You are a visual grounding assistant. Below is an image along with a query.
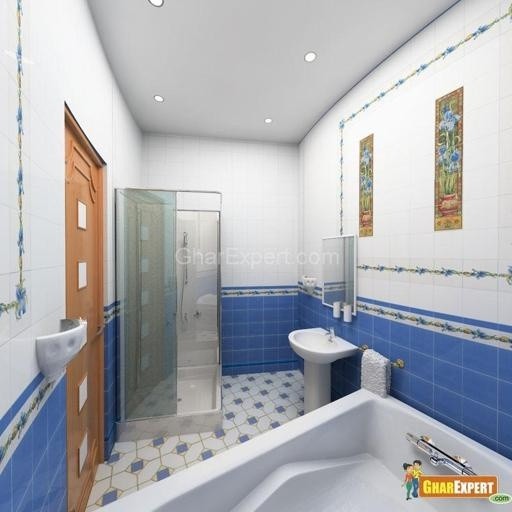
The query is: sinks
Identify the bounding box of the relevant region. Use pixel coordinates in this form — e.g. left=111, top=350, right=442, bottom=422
left=287, top=327, right=359, bottom=365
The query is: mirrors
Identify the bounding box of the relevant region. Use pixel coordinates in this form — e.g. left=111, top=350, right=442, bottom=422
left=321, top=235, right=358, bottom=317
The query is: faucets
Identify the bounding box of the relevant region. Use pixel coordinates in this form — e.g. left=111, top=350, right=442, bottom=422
left=325, top=326, right=335, bottom=342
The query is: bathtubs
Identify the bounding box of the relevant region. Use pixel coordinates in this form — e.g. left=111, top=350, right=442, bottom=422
left=93, top=389, right=512, bottom=512
left=196, top=293, right=218, bottom=329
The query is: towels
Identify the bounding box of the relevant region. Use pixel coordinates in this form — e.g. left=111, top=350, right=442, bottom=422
left=361, top=349, right=392, bottom=398
